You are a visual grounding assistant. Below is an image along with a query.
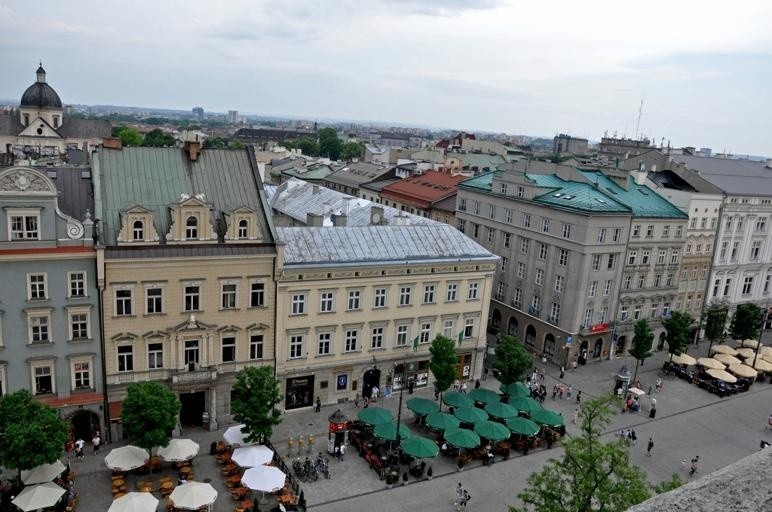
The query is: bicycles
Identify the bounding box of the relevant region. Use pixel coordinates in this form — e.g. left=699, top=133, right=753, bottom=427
left=292, top=451, right=332, bottom=483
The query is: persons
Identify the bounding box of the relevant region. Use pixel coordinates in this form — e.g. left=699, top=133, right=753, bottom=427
left=316, top=352, right=665, bottom=512
left=67, top=435, right=101, bottom=461
left=680, top=453, right=701, bottom=477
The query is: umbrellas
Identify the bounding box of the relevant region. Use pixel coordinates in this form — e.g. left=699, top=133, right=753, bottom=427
left=21, top=458, right=68, bottom=485
left=241, top=465, right=287, bottom=500
left=169, top=480, right=218, bottom=510
left=231, top=444, right=274, bottom=468
left=107, top=491, right=160, bottom=512
left=667, top=335, right=772, bottom=384
left=157, top=438, right=200, bottom=463
left=104, top=445, right=150, bottom=471
left=11, top=481, right=68, bottom=512
left=223, top=423, right=264, bottom=447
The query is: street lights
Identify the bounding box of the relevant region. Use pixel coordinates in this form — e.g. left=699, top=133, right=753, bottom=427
left=393, top=359, right=417, bottom=455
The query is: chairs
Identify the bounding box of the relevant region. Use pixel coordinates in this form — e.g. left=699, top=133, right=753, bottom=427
left=60, top=464, right=80, bottom=512
left=434, top=427, right=560, bottom=466
left=111, top=441, right=307, bottom=512
left=346, top=420, right=432, bottom=485
